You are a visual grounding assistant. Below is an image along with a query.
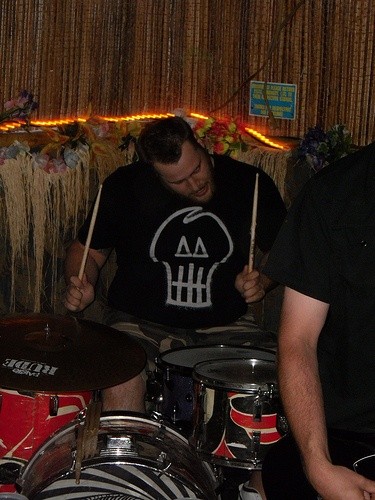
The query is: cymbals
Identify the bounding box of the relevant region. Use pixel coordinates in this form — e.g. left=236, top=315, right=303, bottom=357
left=0, top=313, right=147, bottom=391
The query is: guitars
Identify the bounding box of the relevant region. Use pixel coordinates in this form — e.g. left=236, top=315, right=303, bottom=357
left=261, top=427, right=375, bottom=500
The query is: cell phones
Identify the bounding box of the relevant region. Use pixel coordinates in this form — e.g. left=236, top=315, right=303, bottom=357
left=353, top=454, right=375, bottom=482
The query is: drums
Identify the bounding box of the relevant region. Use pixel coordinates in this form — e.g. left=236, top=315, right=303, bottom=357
left=191, top=358, right=291, bottom=470
left=18, top=410, right=221, bottom=500
left=0, top=389, right=93, bottom=462
left=150, top=345, right=278, bottom=435
left=0, top=456, right=30, bottom=492
left=0, top=492, right=30, bottom=500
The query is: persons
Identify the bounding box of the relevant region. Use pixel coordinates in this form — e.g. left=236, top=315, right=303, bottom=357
left=61, top=116, right=288, bottom=500
left=255, top=140, right=375, bottom=500
